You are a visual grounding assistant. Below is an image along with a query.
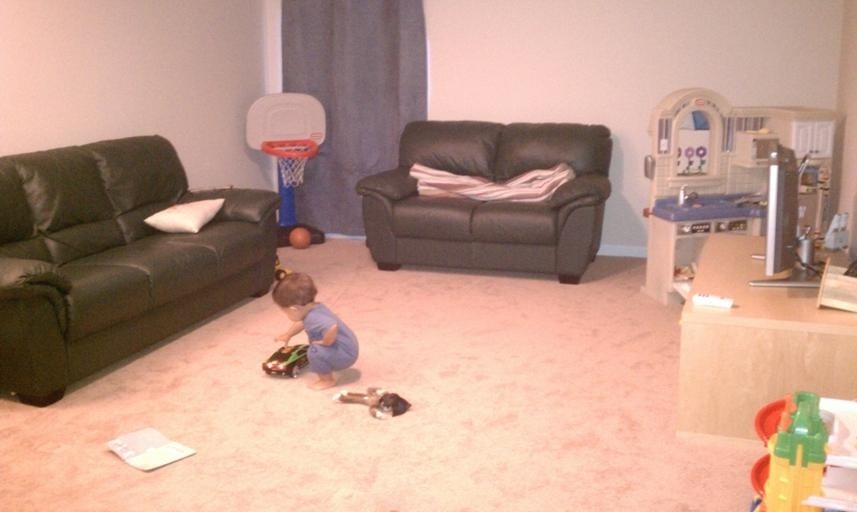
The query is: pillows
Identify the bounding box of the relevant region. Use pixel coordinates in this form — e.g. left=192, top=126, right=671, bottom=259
left=139, top=195, right=228, bottom=235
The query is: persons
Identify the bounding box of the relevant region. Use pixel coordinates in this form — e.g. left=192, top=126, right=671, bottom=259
left=333, top=384, right=411, bottom=420
left=271, top=272, right=360, bottom=391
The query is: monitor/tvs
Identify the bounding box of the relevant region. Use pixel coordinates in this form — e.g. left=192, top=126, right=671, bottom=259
left=749, top=143, right=819, bottom=288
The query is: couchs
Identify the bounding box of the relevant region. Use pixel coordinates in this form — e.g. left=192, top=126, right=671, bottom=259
left=355, top=121, right=614, bottom=284
left=0, top=130, right=283, bottom=408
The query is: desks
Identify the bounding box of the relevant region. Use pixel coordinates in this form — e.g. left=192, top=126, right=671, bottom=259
left=673, top=229, right=856, bottom=445
